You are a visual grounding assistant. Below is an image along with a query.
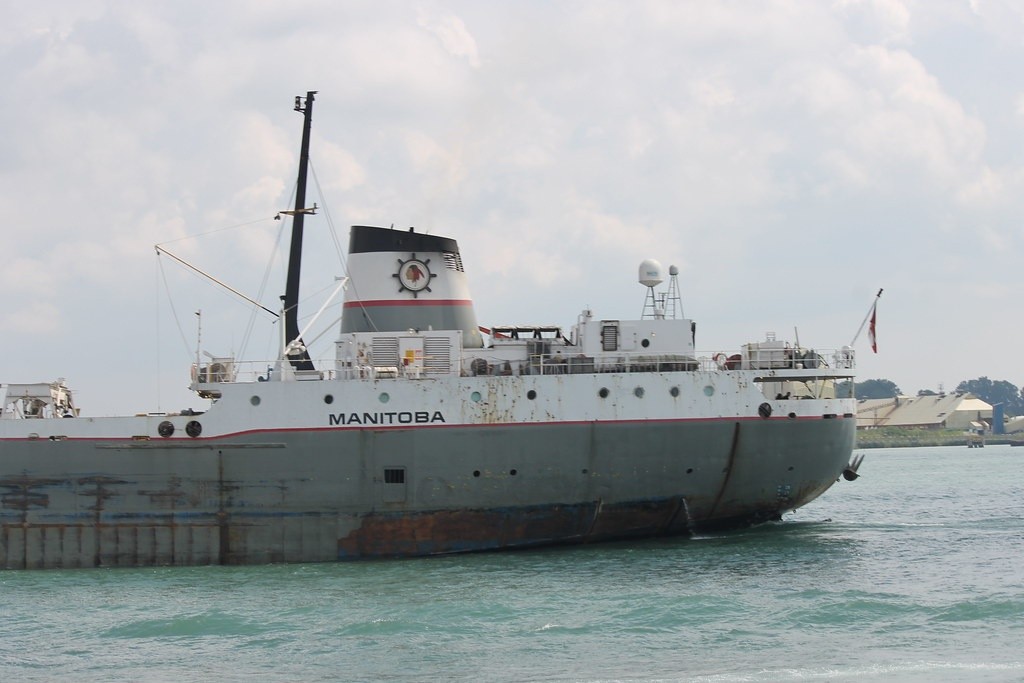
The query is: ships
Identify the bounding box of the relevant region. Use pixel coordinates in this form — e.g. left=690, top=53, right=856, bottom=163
left=0, top=91, right=886, bottom=571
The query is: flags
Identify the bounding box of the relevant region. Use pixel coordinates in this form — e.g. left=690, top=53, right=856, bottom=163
left=868, top=302, right=877, bottom=354
